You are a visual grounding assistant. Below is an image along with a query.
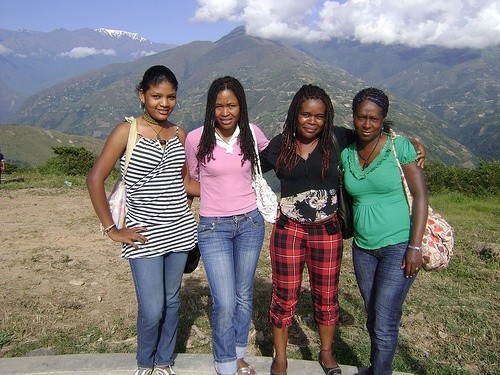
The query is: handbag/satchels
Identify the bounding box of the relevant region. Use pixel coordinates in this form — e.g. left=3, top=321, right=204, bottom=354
left=251, top=174, right=279, bottom=224
left=100, top=179, right=126, bottom=237
left=337, top=170, right=354, bottom=240
left=407, top=197, right=454, bottom=273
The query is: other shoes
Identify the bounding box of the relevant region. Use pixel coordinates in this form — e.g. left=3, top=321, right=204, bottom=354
left=152, top=365, right=176, bottom=375
left=130, top=369, right=153, bottom=375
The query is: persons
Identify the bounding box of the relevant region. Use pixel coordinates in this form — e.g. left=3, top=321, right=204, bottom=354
left=342, top=88, right=429, bottom=375
left=254, top=84, right=425, bottom=375
left=184, top=75, right=270, bottom=375
left=87, top=65, right=199, bottom=375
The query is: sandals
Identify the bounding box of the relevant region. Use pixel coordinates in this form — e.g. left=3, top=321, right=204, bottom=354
left=233, top=364, right=253, bottom=375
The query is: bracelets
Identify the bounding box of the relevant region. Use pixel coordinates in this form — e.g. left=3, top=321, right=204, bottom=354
left=408, top=245, right=424, bottom=251
left=103, top=224, right=116, bottom=232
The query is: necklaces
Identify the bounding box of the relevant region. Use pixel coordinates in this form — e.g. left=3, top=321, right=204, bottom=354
left=146, top=121, right=165, bottom=140
left=356, top=132, right=382, bottom=170
left=142, top=112, right=159, bottom=124
left=301, top=144, right=316, bottom=156
left=215, top=128, right=233, bottom=143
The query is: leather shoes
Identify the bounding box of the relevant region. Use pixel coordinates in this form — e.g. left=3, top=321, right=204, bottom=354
left=271, top=359, right=288, bottom=375
left=318, top=352, right=342, bottom=375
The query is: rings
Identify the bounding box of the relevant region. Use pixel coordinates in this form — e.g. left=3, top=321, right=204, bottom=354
left=416, top=268, right=418, bottom=270
